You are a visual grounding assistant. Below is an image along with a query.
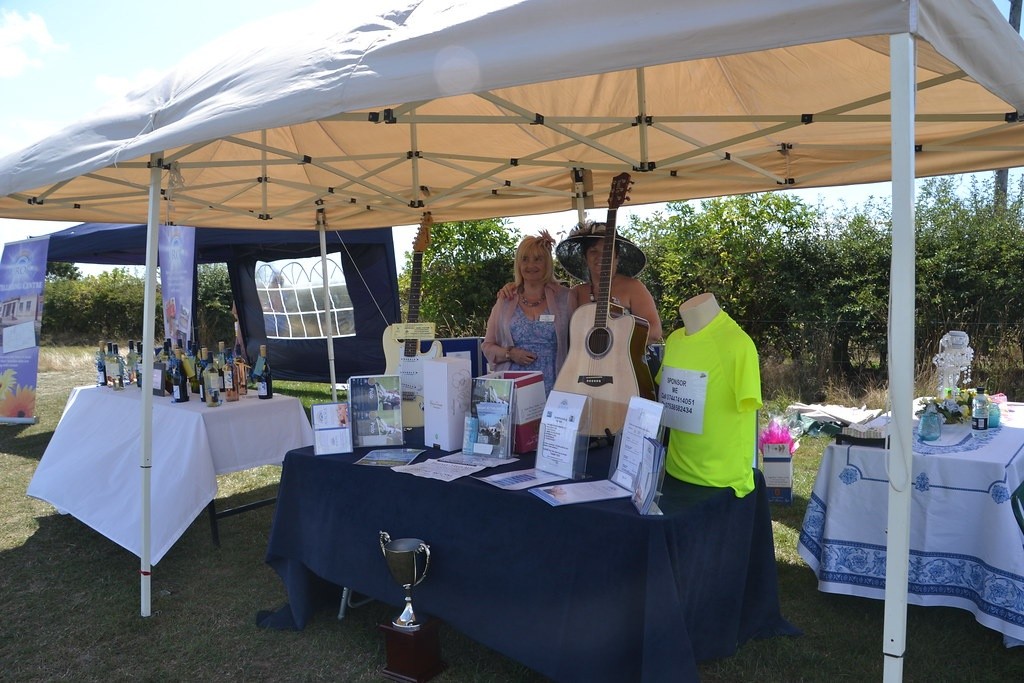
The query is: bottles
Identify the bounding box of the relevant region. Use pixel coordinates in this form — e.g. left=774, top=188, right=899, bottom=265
left=98, top=340, right=272, bottom=408
left=972, top=387, right=988, bottom=437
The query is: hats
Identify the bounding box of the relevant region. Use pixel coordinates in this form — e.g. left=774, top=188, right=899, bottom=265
left=555, top=220, right=646, bottom=282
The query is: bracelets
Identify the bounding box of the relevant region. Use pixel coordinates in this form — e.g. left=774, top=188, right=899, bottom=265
left=505, top=346, right=514, bottom=361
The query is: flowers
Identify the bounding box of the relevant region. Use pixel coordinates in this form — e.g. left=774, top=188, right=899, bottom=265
left=919, top=389, right=978, bottom=422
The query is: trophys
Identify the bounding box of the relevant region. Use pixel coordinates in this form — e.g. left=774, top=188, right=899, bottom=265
left=379, top=531, right=445, bottom=683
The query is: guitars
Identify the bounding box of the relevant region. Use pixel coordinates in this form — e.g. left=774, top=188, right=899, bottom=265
left=548, top=171, right=657, bottom=442
left=381, top=208, right=443, bottom=431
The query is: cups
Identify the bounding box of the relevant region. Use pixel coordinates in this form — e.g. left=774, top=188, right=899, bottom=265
left=988, top=403, right=1000, bottom=428
left=918, top=415, right=941, bottom=440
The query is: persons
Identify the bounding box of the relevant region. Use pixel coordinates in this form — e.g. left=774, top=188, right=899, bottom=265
left=556, top=222, right=662, bottom=345
left=480, top=236, right=579, bottom=401
left=0, top=0, right=1024, bottom=683
left=654, top=293, right=763, bottom=503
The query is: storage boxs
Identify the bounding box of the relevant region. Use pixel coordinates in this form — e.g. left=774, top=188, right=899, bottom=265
left=422, top=358, right=472, bottom=451
left=760, top=438, right=800, bottom=505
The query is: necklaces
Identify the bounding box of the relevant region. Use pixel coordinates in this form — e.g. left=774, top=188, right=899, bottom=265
left=590, top=283, right=597, bottom=303
left=518, top=284, right=546, bottom=307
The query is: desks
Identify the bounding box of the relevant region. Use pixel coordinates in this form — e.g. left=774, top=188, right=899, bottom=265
left=71, top=384, right=306, bottom=544
left=797, top=417, right=1024, bottom=648
left=269, top=427, right=798, bottom=683
